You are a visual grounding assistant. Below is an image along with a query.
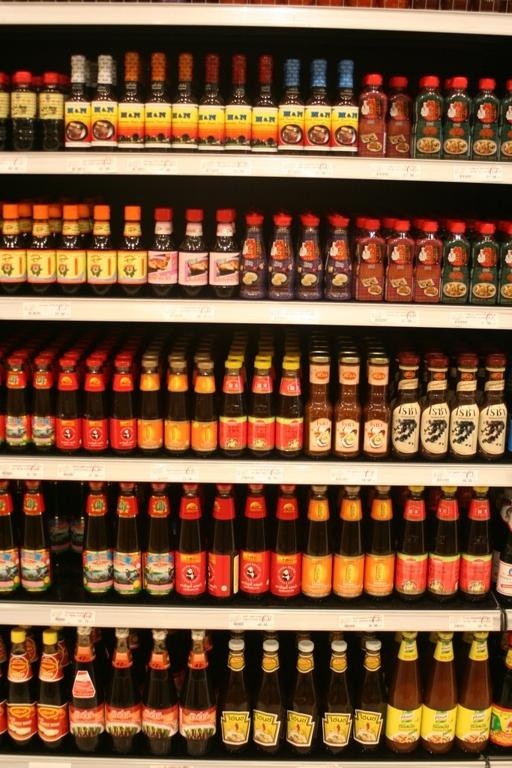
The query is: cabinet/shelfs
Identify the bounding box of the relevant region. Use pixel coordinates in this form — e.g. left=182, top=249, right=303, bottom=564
left=0, top=0, right=512, bottom=768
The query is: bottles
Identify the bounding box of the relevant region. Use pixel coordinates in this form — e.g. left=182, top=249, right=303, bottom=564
left=0, top=0, right=512, bottom=761
